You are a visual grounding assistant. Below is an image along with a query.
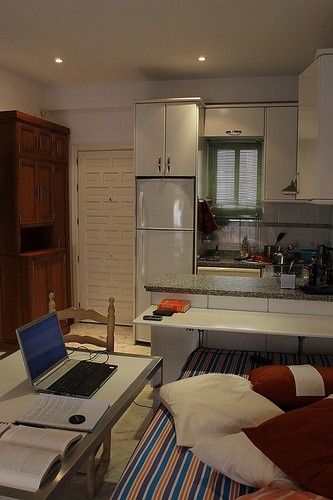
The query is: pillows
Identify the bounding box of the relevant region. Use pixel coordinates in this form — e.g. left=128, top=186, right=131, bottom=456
left=151, top=362, right=333, bottom=500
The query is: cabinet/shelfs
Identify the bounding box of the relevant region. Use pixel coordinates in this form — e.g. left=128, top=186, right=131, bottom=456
left=295, top=53, right=333, bottom=206
left=261, top=103, right=296, bottom=204
left=133, top=96, right=206, bottom=177
left=0, top=110, right=71, bottom=353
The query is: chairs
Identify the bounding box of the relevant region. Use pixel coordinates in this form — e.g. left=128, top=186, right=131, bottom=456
left=48, top=292, right=115, bottom=500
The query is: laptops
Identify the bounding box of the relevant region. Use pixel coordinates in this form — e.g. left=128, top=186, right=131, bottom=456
left=15, top=310, right=119, bottom=400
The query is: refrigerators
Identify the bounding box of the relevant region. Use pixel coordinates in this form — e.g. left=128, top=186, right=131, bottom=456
left=133, top=177, right=196, bottom=347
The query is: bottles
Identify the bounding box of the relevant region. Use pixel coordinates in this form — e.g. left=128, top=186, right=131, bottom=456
left=242, top=237, right=248, bottom=251
left=308, top=255, right=317, bottom=286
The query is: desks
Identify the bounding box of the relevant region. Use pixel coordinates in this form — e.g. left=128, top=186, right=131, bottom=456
left=0, top=345, right=163, bottom=500
left=132, top=304, right=333, bottom=355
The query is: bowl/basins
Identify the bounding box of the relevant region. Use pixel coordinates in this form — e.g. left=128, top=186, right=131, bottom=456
left=297, top=248, right=318, bottom=259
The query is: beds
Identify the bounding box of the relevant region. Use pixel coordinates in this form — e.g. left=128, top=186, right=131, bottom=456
left=108, top=347, right=333, bottom=500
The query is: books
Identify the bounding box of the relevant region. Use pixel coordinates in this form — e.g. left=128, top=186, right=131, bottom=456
left=11, top=393, right=110, bottom=432
left=0, top=420, right=11, bottom=435
left=157, top=299, right=191, bottom=313
left=0, top=424, right=83, bottom=492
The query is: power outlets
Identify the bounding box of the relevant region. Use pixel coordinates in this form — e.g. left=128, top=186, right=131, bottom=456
left=310, top=240, right=317, bottom=248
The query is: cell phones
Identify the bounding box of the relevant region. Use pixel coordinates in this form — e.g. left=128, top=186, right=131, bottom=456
left=143, top=315, right=162, bottom=321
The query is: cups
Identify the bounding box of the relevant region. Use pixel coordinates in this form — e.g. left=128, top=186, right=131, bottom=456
left=264, top=245, right=274, bottom=258
